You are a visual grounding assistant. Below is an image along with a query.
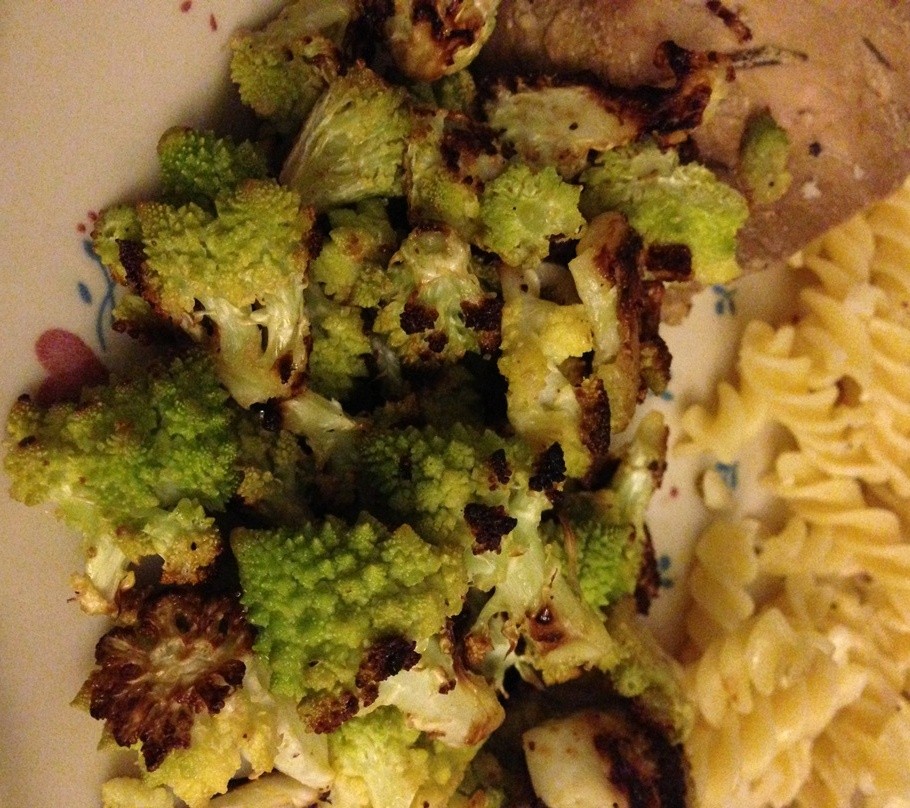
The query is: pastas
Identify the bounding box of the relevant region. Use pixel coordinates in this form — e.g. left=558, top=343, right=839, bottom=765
left=653, top=160, right=910, bottom=808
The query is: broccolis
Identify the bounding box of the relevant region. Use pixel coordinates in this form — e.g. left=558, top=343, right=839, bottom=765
left=0, top=0, right=793, bottom=808
